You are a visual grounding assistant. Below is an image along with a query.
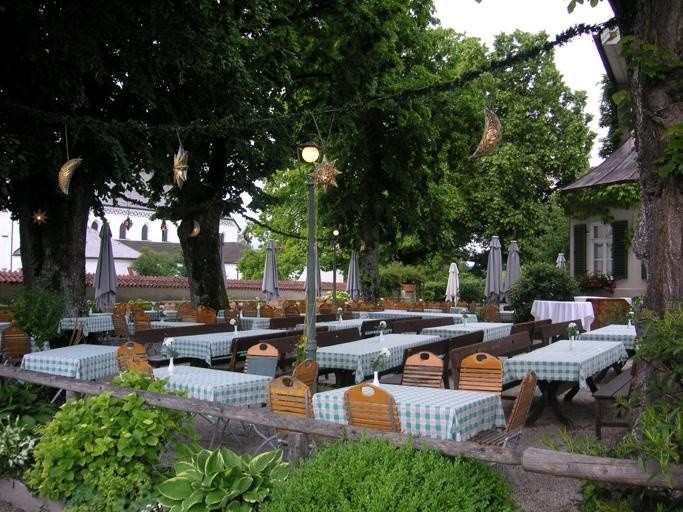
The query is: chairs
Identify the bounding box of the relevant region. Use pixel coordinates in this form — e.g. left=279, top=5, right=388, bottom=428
left=111, top=296, right=500, bottom=337
left=0, top=309, right=31, bottom=364
left=115, top=341, right=320, bottom=442
left=344, top=351, right=538, bottom=452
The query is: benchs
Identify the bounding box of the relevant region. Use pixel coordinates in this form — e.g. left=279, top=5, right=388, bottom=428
left=137, top=312, right=484, bottom=387
left=447, top=319, right=646, bottom=441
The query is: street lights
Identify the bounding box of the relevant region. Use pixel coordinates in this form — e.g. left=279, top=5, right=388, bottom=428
left=331, top=225, right=339, bottom=305
left=296, top=132, right=324, bottom=394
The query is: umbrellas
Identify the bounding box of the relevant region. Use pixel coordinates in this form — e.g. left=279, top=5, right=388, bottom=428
left=302, top=246, right=324, bottom=302
left=94, top=224, right=116, bottom=313
left=260, top=240, right=281, bottom=304
left=216, top=233, right=229, bottom=287
left=483, top=235, right=505, bottom=313
left=344, top=250, right=360, bottom=301
left=555, top=252, right=565, bottom=271
left=445, top=262, right=460, bottom=307
left=505, top=239, right=523, bottom=294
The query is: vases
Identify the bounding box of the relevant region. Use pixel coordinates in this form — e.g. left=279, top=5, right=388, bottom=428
left=367, top=347, right=389, bottom=387
left=375, top=323, right=385, bottom=340
left=229, top=317, right=239, bottom=334
left=335, top=306, right=344, bottom=323
left=157, top=310, right=165, bottom=324
left=164, top=344, right=179, bottom=373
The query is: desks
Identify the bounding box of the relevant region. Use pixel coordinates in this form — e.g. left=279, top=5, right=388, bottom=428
left=216, top=316, right=272, bottom=330
left=149, top=322, right=206, bottom=329
left=163, top=329, right=294, bottom=367
left=296, top=318, right=393, bottom=336
left=422, top=322, right=514, bottom=343
left=310, top=382, right=507, bottom=443
left=0, top=322, right=51, bottom=352
left=19, top=344, right=120, bottom=407
left=316, top=333, right=444, bottom=384
left=57, top=313, right=130, bottom=344
